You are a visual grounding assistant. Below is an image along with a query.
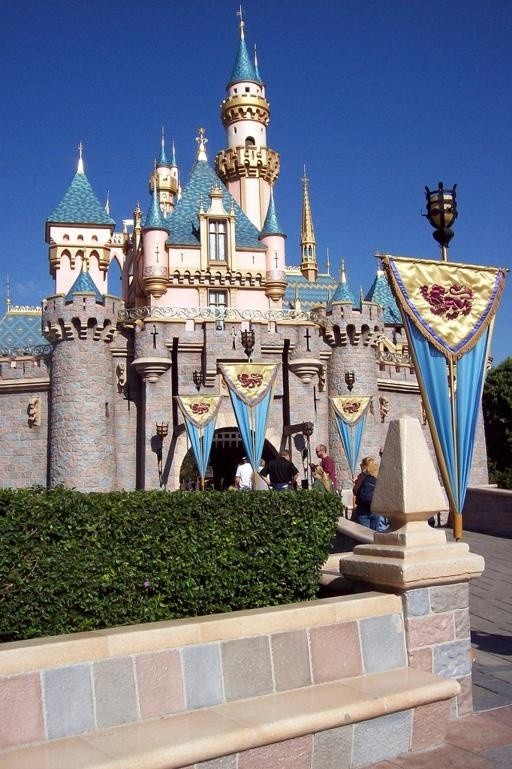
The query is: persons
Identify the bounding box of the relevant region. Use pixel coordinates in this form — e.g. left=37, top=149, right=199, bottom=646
left=309, top=443, right=338, bottom=492
left=259, top=449, right=300, bottom=491
left=254, top=458, right=270, bottom=490
left=308, top=464, right=332, bottom=492
left=234, top=455, right=253, bottom=489
left=351, top=456, right=380, bottom=530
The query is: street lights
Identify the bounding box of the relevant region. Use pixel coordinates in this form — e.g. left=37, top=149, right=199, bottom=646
left=237, top=326, right=260, bottom=491
left=341, top=370, right=357, bottom=508
left=417, top=178, right=464, bottom=541
left=192, top=368, right=207, bottom=492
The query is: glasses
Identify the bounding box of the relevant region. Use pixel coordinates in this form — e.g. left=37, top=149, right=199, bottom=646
left=316, top=451, right=319, bottom=454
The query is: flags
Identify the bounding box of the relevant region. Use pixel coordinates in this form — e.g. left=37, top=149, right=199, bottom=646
left=176, top=394, right=223, bottom=480
left=329, top=394, right=374, bottom=475
left=382, top=254, right=507, bottom=513
left=219, top=361, right=279, bottom=473
